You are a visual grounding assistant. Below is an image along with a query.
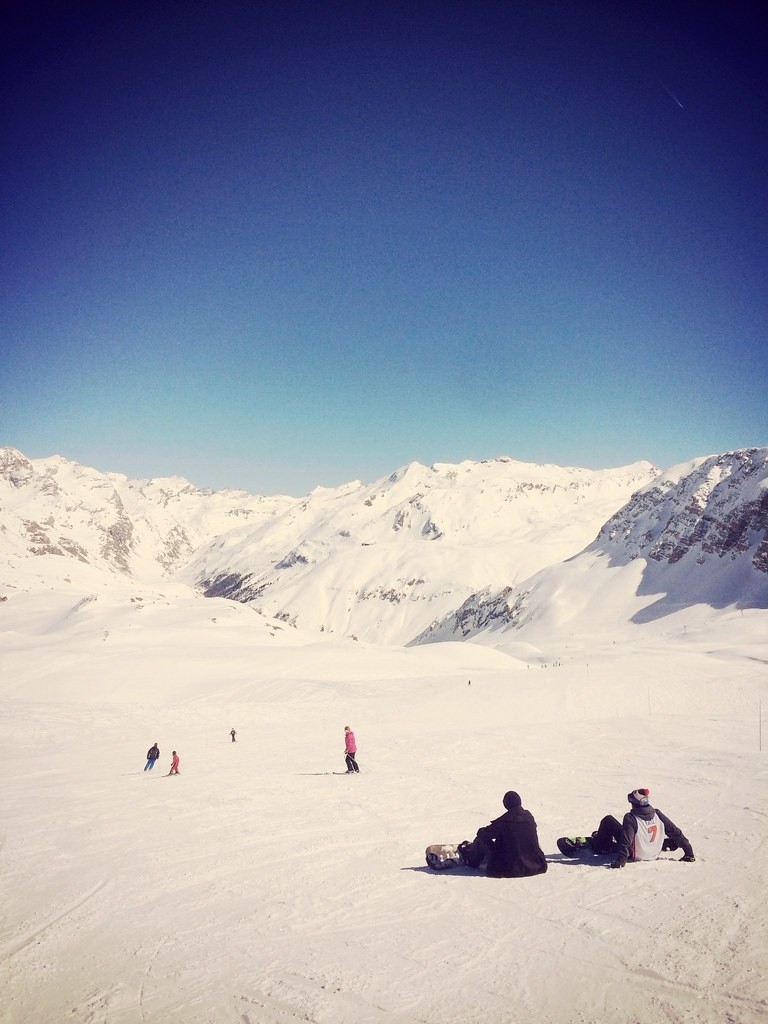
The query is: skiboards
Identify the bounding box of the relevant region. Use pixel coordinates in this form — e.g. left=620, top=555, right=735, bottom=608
left=327, top=772, right=359, bottom=775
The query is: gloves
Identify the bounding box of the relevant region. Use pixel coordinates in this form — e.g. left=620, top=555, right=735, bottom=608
left=678, top=855, right=696, bottom=862
left=610, top=857, right=627, bottom=868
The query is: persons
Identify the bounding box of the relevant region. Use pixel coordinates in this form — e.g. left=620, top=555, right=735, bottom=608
left=229, top=727, right=237, bottom=742
left=593, top=788, right=695, bottom=870
left=458, top=790, right=547, bottom=878
left=344, top=725, right=360, bottom=774
left=143, top=743, right=160, bottom=771
left=167, top=750, right=179, bottom=776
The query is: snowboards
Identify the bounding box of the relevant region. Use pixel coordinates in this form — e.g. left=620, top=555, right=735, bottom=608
left=426, top=844, right=476, bottom=869
left=558, top=837, right=595, bottom=859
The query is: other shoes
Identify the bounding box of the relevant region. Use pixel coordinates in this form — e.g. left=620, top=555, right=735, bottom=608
left=354, top=769, right=359, bottom=772
left=346, top=769, right=355, bottom=773
left=584, top=830, right=614, bottom=854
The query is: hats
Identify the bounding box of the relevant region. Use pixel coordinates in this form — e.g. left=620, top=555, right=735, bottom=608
left=627, top=789, right=649, bottom=806
left=503, top=791, right=522, bottom=810
left=345, top=726, right=350, bottom=730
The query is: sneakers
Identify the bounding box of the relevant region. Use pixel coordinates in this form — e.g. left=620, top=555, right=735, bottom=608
left=458, top=840, right=480, bottom=868
